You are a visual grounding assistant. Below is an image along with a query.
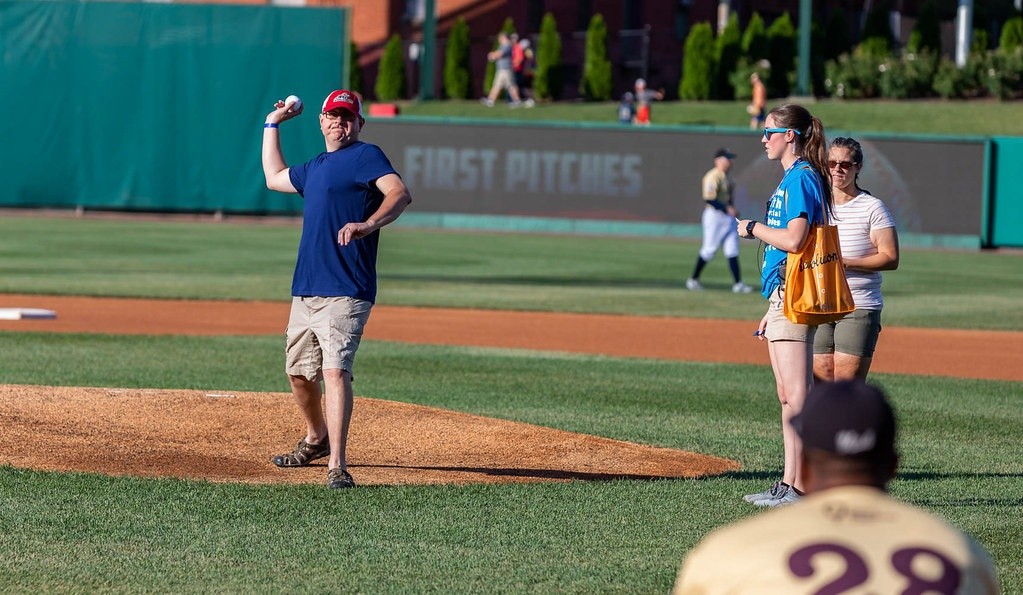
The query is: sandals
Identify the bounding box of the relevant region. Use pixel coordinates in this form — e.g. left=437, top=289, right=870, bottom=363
left=327, top=468, right=353, bottom=489
left=272, top=434, right=330, bottom=468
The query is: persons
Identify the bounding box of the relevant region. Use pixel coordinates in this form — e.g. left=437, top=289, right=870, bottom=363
left=262, top=91, right=412, bottom=489
left=749, top=72, right=767, bottom=128
left=483, top=30, right=536, bottom=108
left=814, top=137, right=899, bottom=384
left=738, top=104, right=840, bottom=506
left=672, top=377, right=1000, bottom=595
left=687, top=150, right=753, bottom=293
left=618, top=78, right=662, bottom=126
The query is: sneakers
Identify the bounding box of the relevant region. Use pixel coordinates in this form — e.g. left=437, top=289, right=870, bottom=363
left=742, top=480, right=788, bottom=502
left=754, top=486, right=803, bottom=507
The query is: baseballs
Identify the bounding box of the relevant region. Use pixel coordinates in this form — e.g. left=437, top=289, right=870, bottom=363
left=285, top=96, right=301, bottom=111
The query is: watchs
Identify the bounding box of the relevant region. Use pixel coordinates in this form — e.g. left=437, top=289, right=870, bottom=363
left=747, top=221, right=759, bottom=237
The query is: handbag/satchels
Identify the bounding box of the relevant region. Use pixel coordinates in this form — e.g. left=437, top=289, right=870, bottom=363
left=783, top=166, right=856, bottom=325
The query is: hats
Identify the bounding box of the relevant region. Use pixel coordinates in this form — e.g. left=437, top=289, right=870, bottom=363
left=714, top=148, right=737, bottom=160
left=322, top=90, right=362, bottom=117
left=789, top=381, right=898, bottom=464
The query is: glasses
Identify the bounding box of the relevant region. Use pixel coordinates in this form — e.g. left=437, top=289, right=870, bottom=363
left=828, top=161, right=857, bottom=169
left=764, top=128, right=801, bottom=139
left=323, top=111, right=359, bottom=121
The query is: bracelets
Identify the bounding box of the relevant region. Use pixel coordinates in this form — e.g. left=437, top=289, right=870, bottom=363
left=264, top=122, right=279, bottom=128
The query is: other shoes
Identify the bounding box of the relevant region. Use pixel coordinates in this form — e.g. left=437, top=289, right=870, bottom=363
left=686, top=279, right=702, bottom=290
left=731, top=283, right=754, bottom=293
